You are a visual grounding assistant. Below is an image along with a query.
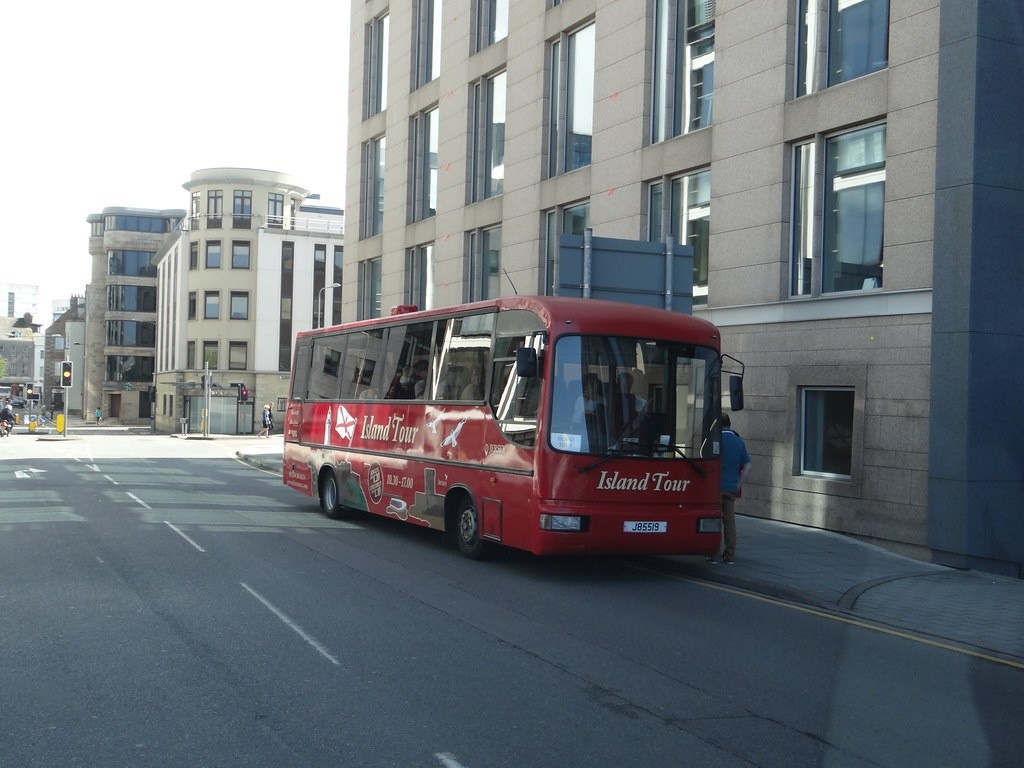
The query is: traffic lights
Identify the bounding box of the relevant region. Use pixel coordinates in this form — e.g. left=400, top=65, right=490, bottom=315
left=27, top=384, right=33, bottom=399
left=243, top=390, right=247, bottom=400
left=60, top=361, right=73, bottom=388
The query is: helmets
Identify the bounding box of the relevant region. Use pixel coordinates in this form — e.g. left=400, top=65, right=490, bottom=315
left=5, top=405, right=12, bottom=411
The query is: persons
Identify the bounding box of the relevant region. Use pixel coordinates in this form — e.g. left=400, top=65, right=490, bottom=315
left=703, top=412, right=750, bottom=566
left=0, top=404, right=15, bottom=432
left=95, top=407, right=102, bottom=425
left=257, top=402, right=275, bottom=438
left=359, top=358, right=484, bottom=400
left=568, top=370, right=647, bottom=439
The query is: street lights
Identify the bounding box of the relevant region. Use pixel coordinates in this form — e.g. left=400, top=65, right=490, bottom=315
left=318, top=283, right=341, bottom=329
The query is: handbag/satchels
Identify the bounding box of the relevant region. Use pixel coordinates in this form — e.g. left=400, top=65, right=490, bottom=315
left=99, top=417, right=102, bottom=422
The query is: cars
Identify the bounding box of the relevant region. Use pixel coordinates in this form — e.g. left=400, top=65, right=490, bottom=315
left=10, top=397, right=24, bottom=406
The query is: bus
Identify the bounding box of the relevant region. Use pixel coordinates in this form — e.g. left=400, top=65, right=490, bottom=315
left=0, top=387, right=11, bottom=398
left=282, top=296, right=744, bottom=559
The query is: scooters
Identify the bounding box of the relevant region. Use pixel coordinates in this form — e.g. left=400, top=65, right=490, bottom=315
left=0, top=418, right=16, bottom=437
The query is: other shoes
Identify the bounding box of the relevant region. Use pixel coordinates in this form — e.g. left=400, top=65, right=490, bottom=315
left=266, top=436, right=270, bottom=439
left=704, top=557, right=719, bottom=564
left=257, top=434, right=261, bottom=438
left=720, top=558, right=734, bottom=564
